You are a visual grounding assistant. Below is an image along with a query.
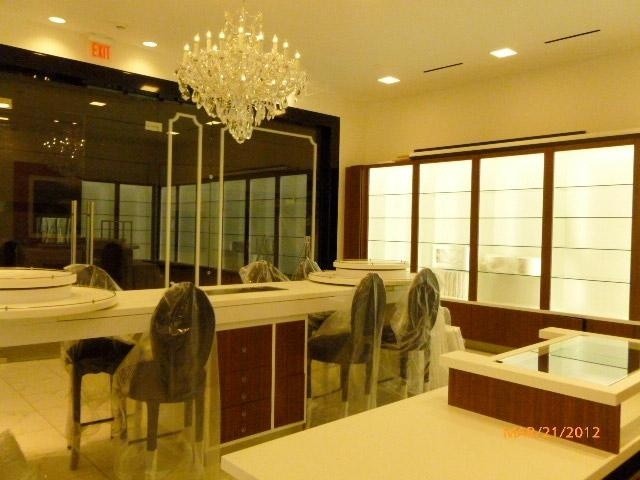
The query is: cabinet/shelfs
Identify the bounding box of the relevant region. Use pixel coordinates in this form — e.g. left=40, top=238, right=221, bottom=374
left=214, top=320, right=305, bottom=444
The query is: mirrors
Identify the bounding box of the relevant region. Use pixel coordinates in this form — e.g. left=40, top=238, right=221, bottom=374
left=1, top=44, right=341, bottom=285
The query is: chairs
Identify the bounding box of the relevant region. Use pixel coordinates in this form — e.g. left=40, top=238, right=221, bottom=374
left=103, top=241, right=129, bottom=293
left=120, top=282, right=217, bottom=480
left=304, top=272, right=382, bottom=423
left=367, top=269, right=442, bottom=402
left=296, top=256, right=341, bottom=341
left=239, top=259, right=291, bottom=288
left=59, top=260, right=137, bottom=468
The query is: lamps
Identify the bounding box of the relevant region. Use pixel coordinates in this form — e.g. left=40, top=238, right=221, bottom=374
left=178, top=2, right=310, bottom=143
